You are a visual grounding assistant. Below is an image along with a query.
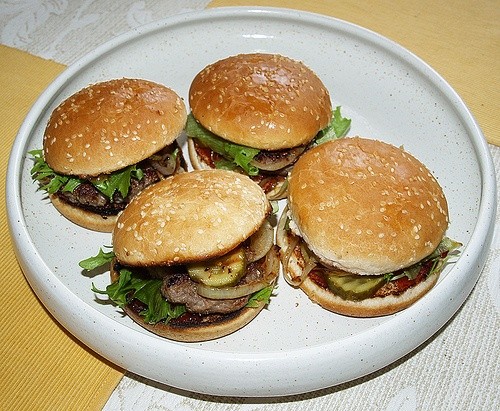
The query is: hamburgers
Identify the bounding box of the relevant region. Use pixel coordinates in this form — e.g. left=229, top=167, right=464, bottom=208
left=182, top=52, right=351, bottom=200
left=28, top=76, right=186, bottom=233
left=78, top=168, right=282, bottom=343
left=276, top=137, right=463, bottom=318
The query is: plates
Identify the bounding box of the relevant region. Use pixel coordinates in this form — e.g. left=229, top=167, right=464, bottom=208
left=6, top=5, right=497, bottom=399
